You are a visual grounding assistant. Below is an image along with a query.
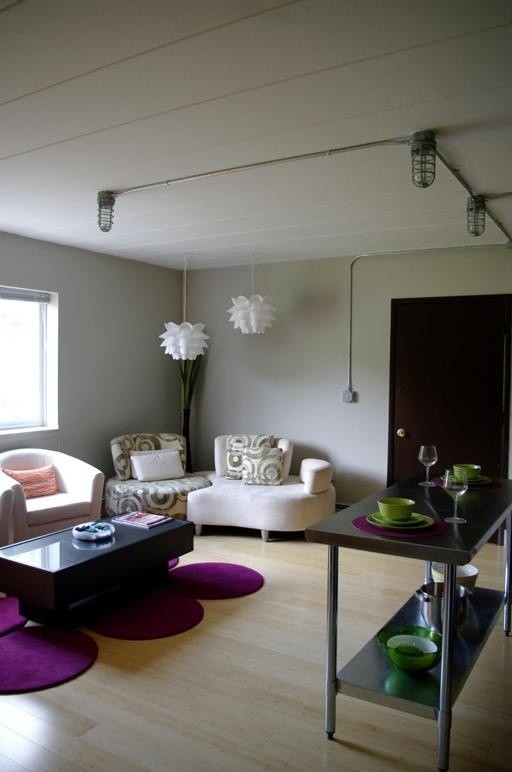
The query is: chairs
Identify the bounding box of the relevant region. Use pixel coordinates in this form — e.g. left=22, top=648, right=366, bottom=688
left=105, top=432, right=211, bottom=522
left=1, top=448, right=105, bottom=543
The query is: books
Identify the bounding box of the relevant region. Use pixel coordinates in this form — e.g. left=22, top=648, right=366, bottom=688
left=111, top=511, right=173, bottom=529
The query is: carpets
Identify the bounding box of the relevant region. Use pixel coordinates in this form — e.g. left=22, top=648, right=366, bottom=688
left=0, top=557, right=263, bottom=695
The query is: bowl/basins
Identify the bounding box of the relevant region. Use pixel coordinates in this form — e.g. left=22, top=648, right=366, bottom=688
left=453, top=464, right=481, bottom=480
left=376, top=625, right=442, bottom=674
left=71, top=538, right=116, bottom=550
left=383, top=669, right=439, bottom=707
left=377, top=497, right=416, bottom=521
left=72, top=521, right=116, bottom=541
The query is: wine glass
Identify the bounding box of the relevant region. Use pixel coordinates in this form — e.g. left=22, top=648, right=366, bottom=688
left=417, top=445, right=438, bottom=487
left=443, top=470, right=469, bottom=524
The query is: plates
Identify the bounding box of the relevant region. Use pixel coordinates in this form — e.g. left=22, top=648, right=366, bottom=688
left=366, top=512, right=436, bottom=530
left=439, top=473, right=492, bottom=485
left=372, top=512, right=424, bottom=525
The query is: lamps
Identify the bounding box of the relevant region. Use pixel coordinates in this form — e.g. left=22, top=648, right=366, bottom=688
left=97, top=190, right=116, bottom=232
left=467, top=195, right=486, bottom=235
left=227, top=253, right=276, bottom=335
left=158, top=263, right=210, bottom=361
left=409, top=129, right=436, bottom=189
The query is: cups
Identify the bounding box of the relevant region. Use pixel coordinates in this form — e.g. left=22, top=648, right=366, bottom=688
left=431, top=561, right=480, bottom=590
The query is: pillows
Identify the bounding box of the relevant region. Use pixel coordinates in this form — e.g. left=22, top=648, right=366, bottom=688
left=242, top=447, right=283, bottom=485
left=4, top=464, right=58, bottom=499
left=130, top=451, right=185, bottom=481
left=226, top=434, right=274, bottom=479
left=130, top=449, right=174, bottom=479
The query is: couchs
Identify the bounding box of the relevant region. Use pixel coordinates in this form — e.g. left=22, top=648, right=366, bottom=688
left=187, top=434, right=336, bottom=542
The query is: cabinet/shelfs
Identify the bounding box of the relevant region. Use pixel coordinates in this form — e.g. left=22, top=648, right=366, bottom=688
left=306, top=479, right=512, bottom=772
